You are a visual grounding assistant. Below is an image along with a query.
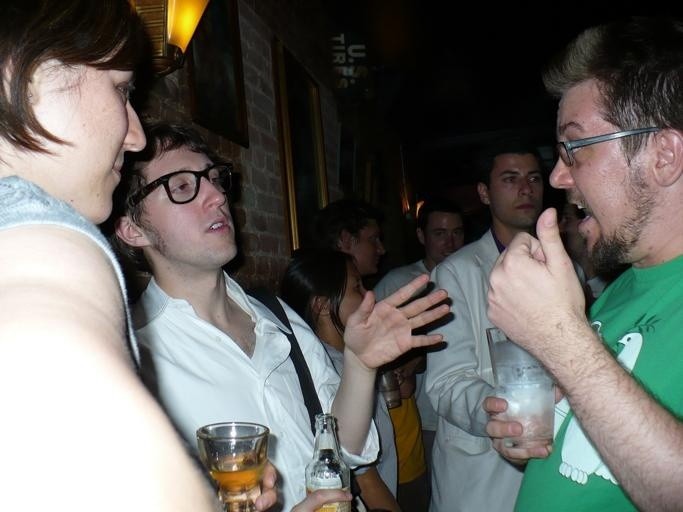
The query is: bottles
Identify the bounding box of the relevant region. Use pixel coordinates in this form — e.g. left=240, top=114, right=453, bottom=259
left=303, top=413, right=351, bottom=512
left=377, top=364, right=402, bottom=408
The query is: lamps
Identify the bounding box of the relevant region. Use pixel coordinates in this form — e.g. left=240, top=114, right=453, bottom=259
left=156, top=0, right=207, bottom=65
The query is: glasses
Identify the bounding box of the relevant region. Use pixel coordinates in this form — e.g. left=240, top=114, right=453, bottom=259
left=555, top=125, right=665, bottom=169
left=122, top=161, right=235, bottom=213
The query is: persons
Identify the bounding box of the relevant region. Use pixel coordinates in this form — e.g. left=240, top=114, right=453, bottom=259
left=481, top=24, right=682, bottom=512
left=0, top=0, right=353, bottom=512
left=96, top=117, right=451, bottom=512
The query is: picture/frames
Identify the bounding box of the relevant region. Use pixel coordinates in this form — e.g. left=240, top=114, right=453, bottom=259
left=189, top=2, right=253, bottom=147
left=270, top=39, right=326, bottom=258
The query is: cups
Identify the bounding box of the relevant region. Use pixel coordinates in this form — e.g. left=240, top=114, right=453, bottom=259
left=197, top=422, right=270, bottom=511
left=485, top=326, right=556, bottom=449
